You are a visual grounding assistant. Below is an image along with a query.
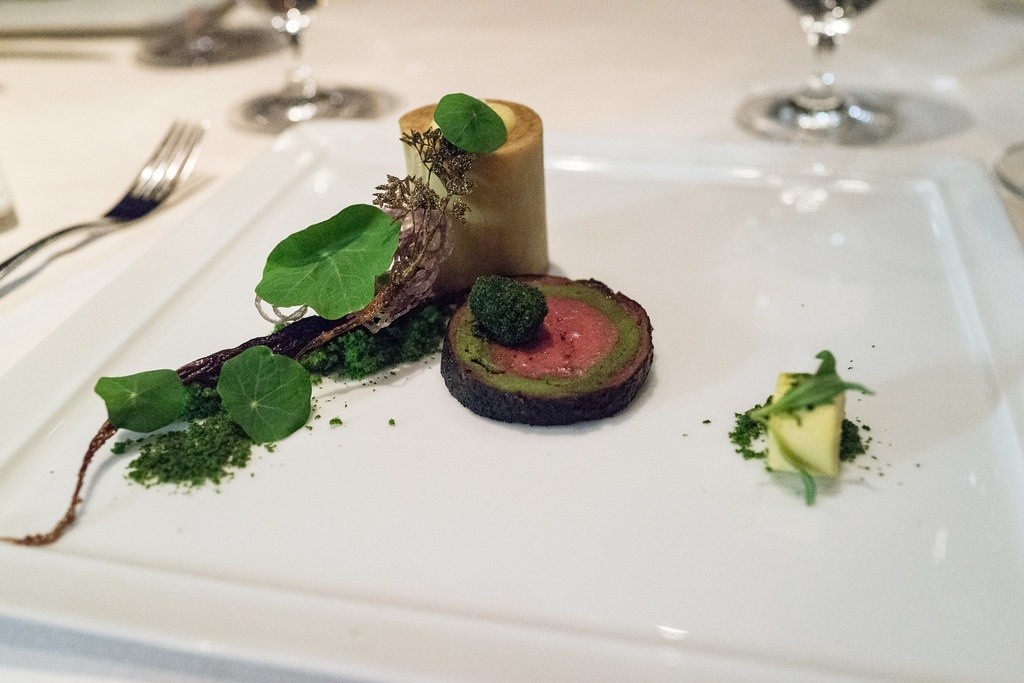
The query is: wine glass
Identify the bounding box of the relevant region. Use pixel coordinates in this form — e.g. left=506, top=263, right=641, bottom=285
left=736, top=0, right=900, bottom=144
left=232, top=0, right=394, bottom=134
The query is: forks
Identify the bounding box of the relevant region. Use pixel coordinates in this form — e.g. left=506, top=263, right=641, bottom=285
left=0, top=117, right=204, bottom=279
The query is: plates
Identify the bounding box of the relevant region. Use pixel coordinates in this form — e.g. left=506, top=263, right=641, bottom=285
left=0, top=119, right=1024, bottom=683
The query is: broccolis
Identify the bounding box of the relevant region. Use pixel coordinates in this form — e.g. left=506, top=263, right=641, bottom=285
left=470, top=273, right=548, bottom=347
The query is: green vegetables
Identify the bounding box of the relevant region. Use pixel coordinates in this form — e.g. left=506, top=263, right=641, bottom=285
left=747, top=350, right=877, bottom=505
left=91, top=93, right=509, bottom=443
